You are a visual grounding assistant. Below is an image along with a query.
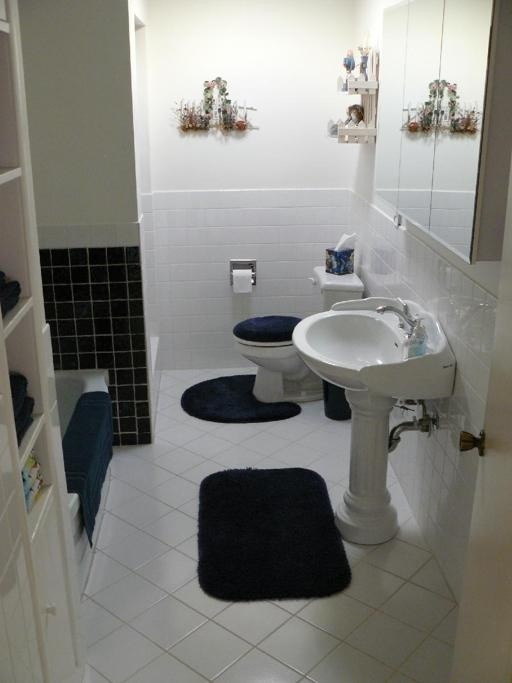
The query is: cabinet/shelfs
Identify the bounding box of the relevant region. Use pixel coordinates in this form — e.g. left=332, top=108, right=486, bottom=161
left=0, top=0, right=94, bottom=681
left=328, top=48, right=380, bottom=145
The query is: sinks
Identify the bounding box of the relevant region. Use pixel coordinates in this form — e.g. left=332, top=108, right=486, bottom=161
left=292, top=299, right=456, bottom=400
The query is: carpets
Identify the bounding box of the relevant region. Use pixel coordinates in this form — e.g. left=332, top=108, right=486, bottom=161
left=195, top=466, right=352, bottom=603
left=179, top=371, right=303, bottom=423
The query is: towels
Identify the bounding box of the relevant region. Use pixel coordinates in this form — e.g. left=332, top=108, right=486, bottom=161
left=0, top=272, right=21, bottom=318
left=63, top=390, right=118, bottom=553
left=10, top=372, right=36, bottom=445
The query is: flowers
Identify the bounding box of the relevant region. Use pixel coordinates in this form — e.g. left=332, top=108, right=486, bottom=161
left=400, top=77, right=481, bottom=137
left=173, top=75, right=259, bottom=135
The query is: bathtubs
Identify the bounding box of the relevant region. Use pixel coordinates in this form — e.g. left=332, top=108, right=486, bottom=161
left=55, top=370, right=110, bottom=512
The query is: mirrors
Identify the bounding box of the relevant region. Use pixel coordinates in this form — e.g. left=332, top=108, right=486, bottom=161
left=373, top=0, right=497, bottom=268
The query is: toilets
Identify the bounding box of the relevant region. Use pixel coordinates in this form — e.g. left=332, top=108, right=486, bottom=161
left=233, top=268, right=364, bottom=404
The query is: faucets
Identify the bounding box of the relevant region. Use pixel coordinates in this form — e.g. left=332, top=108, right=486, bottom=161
left=377, top=306, right=428, bottom=341
